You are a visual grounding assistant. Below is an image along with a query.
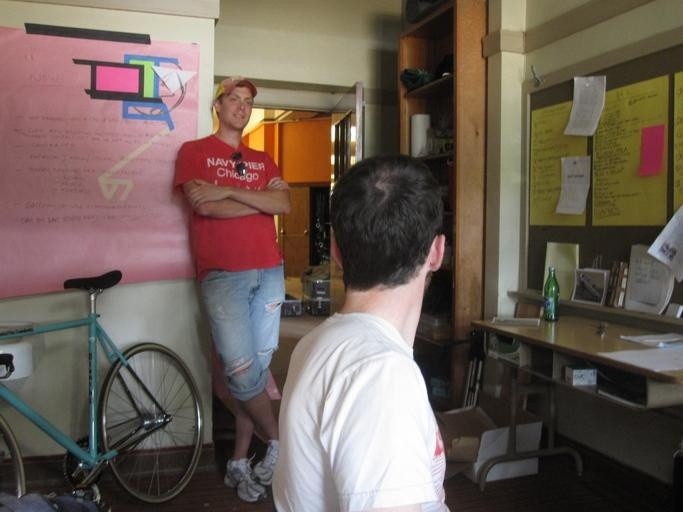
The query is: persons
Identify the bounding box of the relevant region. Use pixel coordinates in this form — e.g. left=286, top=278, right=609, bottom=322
left=272, top=153, right=453, bottom=512
left=173, top=76, right=293, bottom=502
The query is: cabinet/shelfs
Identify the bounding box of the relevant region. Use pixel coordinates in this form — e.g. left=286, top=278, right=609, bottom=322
left=395, top=1, right=488, bottom=410
left=241, top=116, right=333, bottom=187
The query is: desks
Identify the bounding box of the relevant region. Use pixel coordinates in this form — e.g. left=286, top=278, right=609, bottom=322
left=470, top=314, right=683, bottom=510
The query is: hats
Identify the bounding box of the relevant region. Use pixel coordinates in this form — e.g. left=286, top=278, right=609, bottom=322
left=214, top=79, right=257, bottom=100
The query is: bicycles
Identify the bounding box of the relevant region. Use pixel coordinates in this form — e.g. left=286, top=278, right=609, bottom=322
left=0, top=271, right=204, bottom=510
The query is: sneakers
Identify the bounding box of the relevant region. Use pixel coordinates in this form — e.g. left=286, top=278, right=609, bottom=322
left=225, top=452, right=268, bottom=504
left=251, top=440, right=279, bottom=486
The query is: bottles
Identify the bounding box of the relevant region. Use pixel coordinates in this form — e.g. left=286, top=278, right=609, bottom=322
left=543, top=265, right=560, bottom=322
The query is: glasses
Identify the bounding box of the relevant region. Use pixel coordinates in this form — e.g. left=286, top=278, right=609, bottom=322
left=232, top=151, right=247, bottom=176
